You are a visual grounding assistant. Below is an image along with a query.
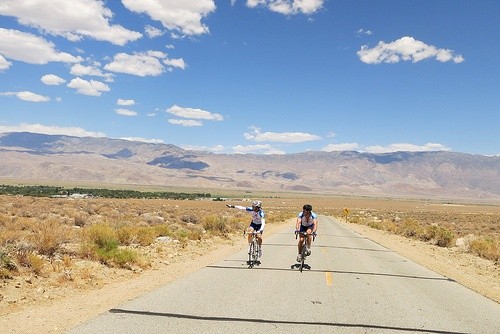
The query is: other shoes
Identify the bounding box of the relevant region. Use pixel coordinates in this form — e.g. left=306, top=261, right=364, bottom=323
left=259, top=249, right=261, bottom=256
left=306, top=247, right=311, bottom=255
left=248, top=247, right=252, bottom=253
left=297, top=254, right=302, bottom=262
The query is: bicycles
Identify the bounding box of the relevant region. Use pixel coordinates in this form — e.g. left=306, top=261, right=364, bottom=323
left=295, top=232, right=317, bottom=273
left=244, top=231, right=262, bottom=268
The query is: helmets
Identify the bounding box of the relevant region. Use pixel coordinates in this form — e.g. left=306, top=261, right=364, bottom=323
left=251, top=200, right=262, bottom=207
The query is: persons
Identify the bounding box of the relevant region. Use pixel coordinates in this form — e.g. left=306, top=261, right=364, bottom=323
left=295, top=204, right=318, bottom=262
left=226, top=200, right=266, bottom=255
